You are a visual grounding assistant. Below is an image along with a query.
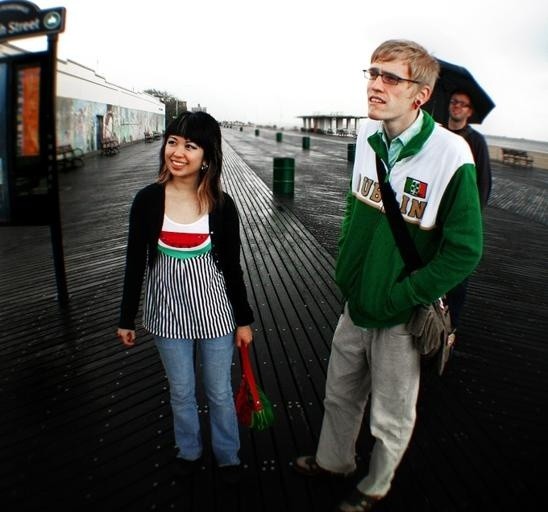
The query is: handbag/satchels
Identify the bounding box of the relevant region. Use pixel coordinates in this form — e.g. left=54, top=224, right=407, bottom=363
left=235, top=341, right=274, bottom=431
left=403, top=294, right=457, bottom=378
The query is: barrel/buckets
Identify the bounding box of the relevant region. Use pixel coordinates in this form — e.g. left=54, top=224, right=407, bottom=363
left=240, top=127, right=356, bottom=163
left=273, top=157, right=294, bottom=198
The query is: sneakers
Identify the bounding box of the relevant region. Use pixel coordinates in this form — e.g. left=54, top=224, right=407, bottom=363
left=174, top=458, right=192, bottom=477
left=220, top=465, right=241, bottom=486
left=291, top=456, right=343, bottom=477
left=335, top=490, right=378, bottom=512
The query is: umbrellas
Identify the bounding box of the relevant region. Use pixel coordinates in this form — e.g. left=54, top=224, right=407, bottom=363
left=422, top=56, right=494, bottom=127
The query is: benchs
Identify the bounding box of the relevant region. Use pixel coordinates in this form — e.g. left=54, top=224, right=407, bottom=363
left=55, top=142, right=86, bottom=170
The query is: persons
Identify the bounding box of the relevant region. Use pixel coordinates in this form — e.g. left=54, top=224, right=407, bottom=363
left=288, top=40, right=482, bottom=511
left=441, top=89, right=493, bottom=347
left=116, top=112, right=255, bottom=488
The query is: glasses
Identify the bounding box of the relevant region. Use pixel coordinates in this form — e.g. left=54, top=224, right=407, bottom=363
left=449, top=98, right=471, bottom=109
left=362, top=69, right=420, bottom=85
